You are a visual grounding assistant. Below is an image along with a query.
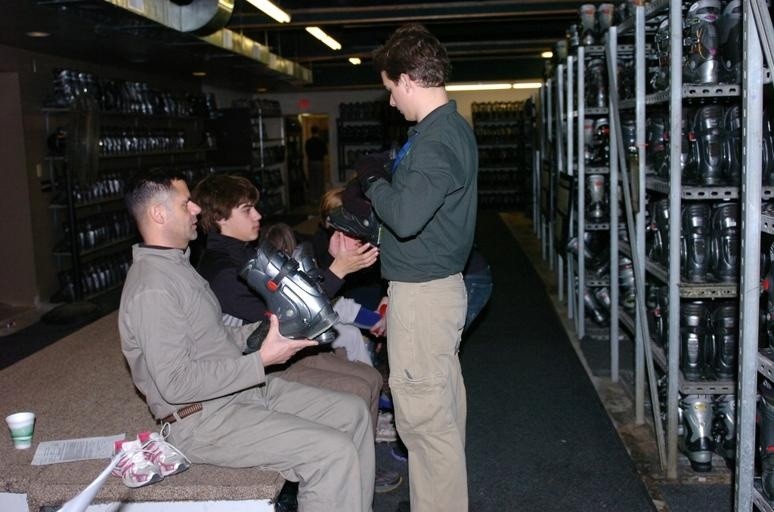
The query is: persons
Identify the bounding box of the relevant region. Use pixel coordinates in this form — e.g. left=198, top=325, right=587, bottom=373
left=191, top=174, right=403, bottom=493
left=117, top=169, right=377, bottom=511
left=342, top=23, right=480, bottom=512
left=305, top=125, right=327, bottom=207
left=255, top=219, right=401, bottom=445
left=314, top=185, right=497, bottom=462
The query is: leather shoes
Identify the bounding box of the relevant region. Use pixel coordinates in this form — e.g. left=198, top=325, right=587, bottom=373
left=374, top=466, right=403, bottom=493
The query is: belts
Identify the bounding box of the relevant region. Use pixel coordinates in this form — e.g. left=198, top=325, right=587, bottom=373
left=157, top=401, right=202, bottom=427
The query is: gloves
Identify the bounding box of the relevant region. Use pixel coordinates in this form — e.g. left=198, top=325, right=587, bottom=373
left=354, top=151, right=385, bottom=183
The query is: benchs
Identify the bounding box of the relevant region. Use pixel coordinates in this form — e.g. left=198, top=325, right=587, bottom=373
left=0, top=307, right=297, bottom=511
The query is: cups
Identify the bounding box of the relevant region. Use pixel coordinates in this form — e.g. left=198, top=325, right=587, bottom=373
left=6, top=412, right=35, bottom=449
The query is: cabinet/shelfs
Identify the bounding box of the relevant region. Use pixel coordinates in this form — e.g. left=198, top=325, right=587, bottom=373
left=17, top=50, right=388, bottom=307
left=469, top=1, right=774, bottom=427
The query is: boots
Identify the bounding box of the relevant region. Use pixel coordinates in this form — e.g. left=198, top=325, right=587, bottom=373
left=238, top=241, right=339, bottom=349
left=327, top=198, right=379, bottom=249
left=471, top=101, right=527, bottom=208
left=46, top=80, right=216, bottom=304
left=527, top=0, right=774, bottom=498
left=291, top=242, right=337, bottom=345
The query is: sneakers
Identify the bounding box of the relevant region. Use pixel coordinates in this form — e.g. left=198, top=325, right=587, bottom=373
left=137, top=421, right=192, bottom=475
left=379, top=394, right=393, bottom=409
left=110, top=438, right=163, bottom=489
left=376, top=409, right=395, bottom=428
left=374, top=424, right=397, bottom=443
